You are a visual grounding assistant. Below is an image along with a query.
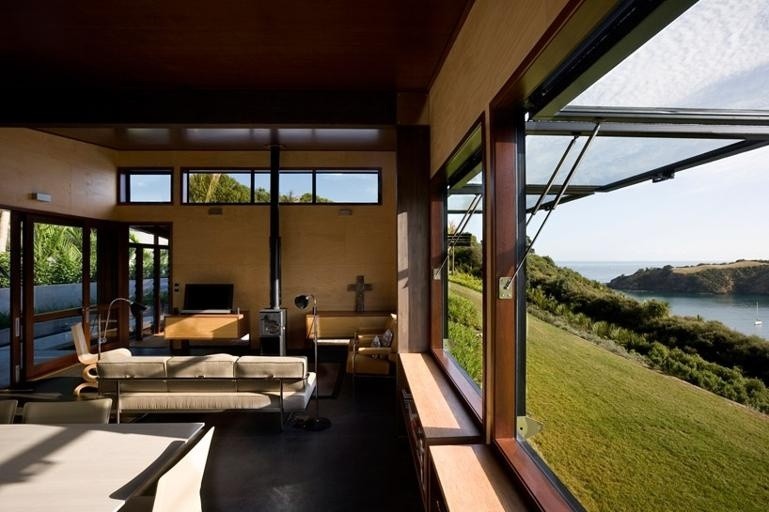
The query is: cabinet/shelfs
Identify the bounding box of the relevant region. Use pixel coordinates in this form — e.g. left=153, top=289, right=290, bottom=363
left=398, top=352, right=483, bottom=502
left=424, top=444, right=538, bottom=511
left=163, top=311, right=251, bottom=357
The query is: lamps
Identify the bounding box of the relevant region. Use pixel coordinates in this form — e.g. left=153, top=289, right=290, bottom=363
left=294, top=294, right=331, bottom=431
left=102, top=299, right=146, bottom=361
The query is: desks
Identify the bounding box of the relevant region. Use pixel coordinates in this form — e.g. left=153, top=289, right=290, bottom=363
left=0, top=424, right=206, bottom=512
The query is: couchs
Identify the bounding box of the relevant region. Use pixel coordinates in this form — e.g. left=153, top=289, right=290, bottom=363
left=97, top=355, right=316, bottom=430
left=305, top=313, right=392, bottom=348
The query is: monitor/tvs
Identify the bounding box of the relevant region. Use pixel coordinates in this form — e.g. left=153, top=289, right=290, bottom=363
left=181, top=284, right=234, bottom=314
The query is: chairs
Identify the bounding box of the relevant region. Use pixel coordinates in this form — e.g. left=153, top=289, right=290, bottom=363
left=71, top=322, right=133, bottom=399
left=131, top=427, right=215, bottom=512
left=0, top=399, right=19, bottom=423
left=21, top=398, right=111, bottom=425
